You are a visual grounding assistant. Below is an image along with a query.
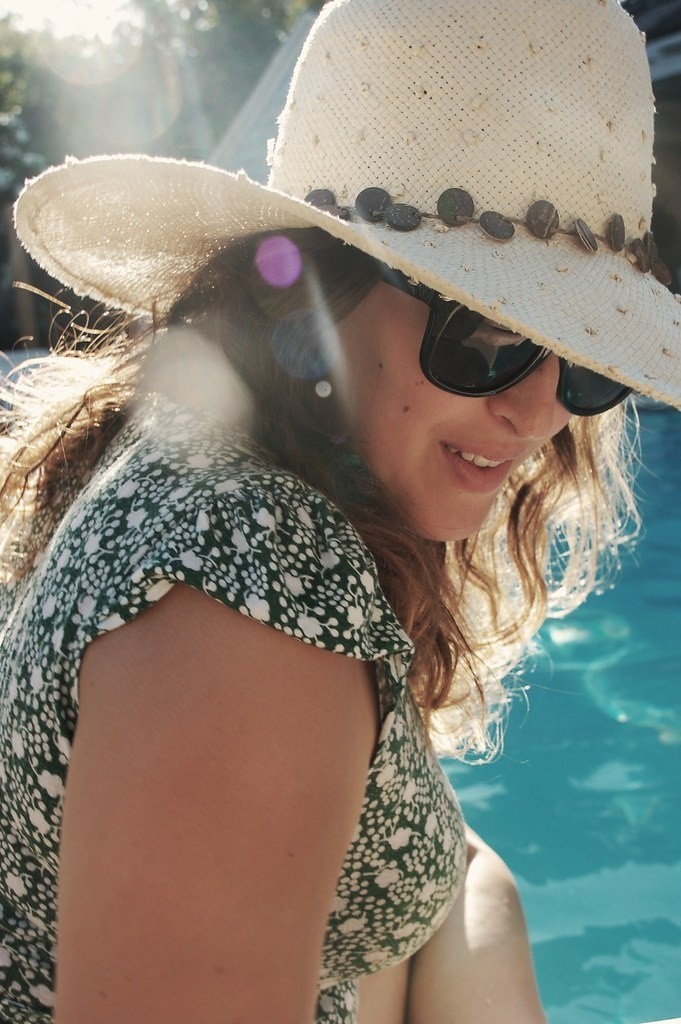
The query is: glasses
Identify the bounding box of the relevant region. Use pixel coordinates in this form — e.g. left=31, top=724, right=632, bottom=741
left=335, top=242, right=634, bottom=417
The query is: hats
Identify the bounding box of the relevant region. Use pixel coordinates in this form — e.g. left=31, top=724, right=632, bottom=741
left=9, top=0, right=681, bottom=412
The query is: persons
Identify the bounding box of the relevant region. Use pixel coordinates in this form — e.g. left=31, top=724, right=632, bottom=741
left=0, top=1, right=681, bottom=1024
